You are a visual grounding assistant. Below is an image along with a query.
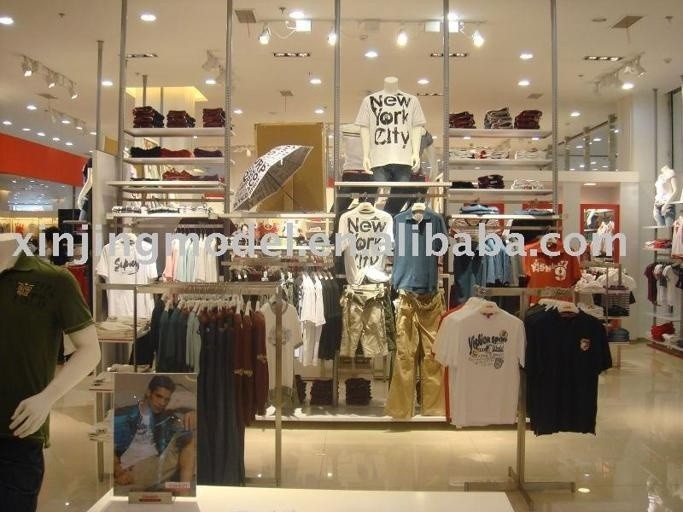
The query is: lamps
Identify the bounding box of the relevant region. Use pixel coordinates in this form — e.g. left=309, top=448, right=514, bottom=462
left=18, top=53, right=80, bottom=101
left=258, top=7, right=485, bottom=47
left=595, top=52, right=648, bottom=94
left=204, top=52, right=226, bottom=87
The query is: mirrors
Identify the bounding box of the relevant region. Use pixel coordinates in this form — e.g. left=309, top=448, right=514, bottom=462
left=580, top=202, right=620, bottom=265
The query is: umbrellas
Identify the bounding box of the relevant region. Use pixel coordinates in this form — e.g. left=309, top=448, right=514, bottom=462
left=230, top=144, right=313, bottom=214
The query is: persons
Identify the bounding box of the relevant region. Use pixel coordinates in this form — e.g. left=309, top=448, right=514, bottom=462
left=112, top=376, right=194, bottom=494
left=75, top=158, right=93, bottom=221
left=0, top=230, right=103, bottom=512
left=41, top=226, right=68, bottom=366
left=652, top=164, right=677, bottom=225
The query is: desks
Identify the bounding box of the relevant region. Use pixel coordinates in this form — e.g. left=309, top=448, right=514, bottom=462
left=87, top=485, right=518, bottom=512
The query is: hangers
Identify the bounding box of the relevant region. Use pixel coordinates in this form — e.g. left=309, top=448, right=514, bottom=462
left=462, top=284, right=505, bottom=317
left=537, top=287, right=582, bottom=314
left=295, top=262, right=336, bottom=282
left=349, top=192, right=428, bottom=214
left=161, top=280, right=292, bottom=316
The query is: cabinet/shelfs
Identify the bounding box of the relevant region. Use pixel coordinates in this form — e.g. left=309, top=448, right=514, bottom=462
left=89, top=284, right=154, bottom=483
left=575, top=265, right=631, bottom=367
left=107, top=126, right=234, bottom=214
left=447, top=127, right=553, bottom=202
left=642, top=225, right=683, bottom=356
